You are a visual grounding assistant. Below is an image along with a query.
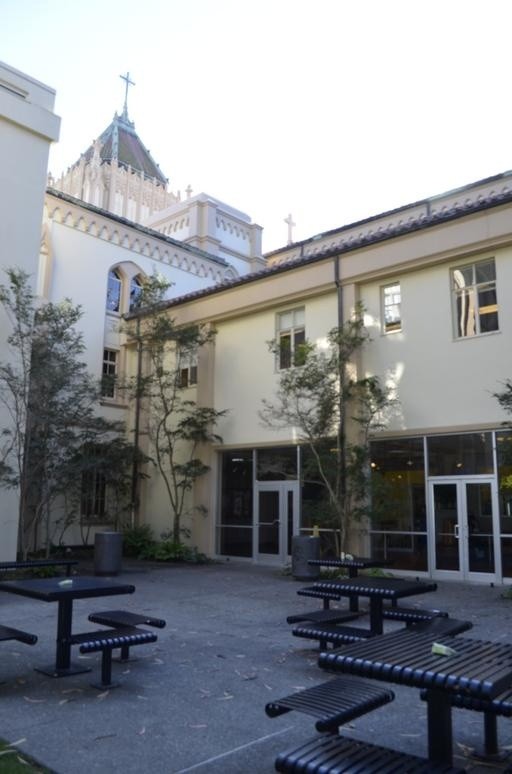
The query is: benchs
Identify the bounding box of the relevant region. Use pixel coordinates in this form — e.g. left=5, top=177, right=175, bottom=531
left=0, top=624, right=37, bottom=645
left=61, top=626, right=157, bottom=690
left=88, top=610, right=166, bottom=663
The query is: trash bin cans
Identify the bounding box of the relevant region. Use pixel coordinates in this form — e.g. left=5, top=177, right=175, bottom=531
left=292, top=535, right=322, bottom=581
left=93, top=531, right=125, bottom=575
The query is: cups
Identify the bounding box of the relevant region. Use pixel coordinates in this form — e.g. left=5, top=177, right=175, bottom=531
left=341, top=551, right=354, bottom=560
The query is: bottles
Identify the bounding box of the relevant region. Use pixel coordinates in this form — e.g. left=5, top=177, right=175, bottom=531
left=313, top=525, right=319, bottom=537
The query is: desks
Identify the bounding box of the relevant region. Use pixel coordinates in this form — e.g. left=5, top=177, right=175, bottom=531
left=0, top=576, right=135, bottom=677
left=308, top=555, right=394, bottom=577
